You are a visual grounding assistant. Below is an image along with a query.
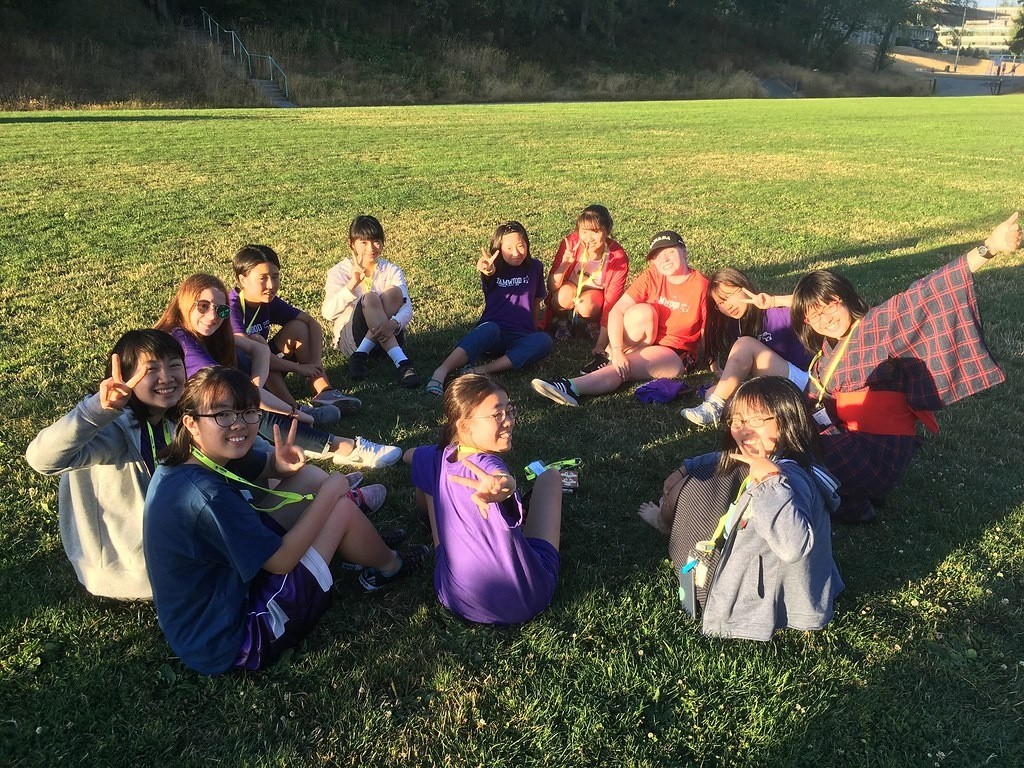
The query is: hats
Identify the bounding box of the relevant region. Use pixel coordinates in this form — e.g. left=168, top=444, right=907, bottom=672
left=646, top=231, right=686, bottom=260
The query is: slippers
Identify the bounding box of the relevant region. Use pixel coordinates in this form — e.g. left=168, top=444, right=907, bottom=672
left=460, top=364, right=475, bottom=376
left=424, top=377, right=444, bottom=398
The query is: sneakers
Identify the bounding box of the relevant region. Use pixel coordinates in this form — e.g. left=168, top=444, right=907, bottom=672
left=300, top=403, right=341, bottom=424
left=580, top=354, right=610, bottom=375
left=530, top=377, right=580, bottom=407
left=394, top=359, right=422, bottom=387
left=348, top=352, right=369, bottom=380
left=345, top=484, right=386, bottom=515
left=679, top=401, right=723, bottom=427
left=359, top=544, right=429, bottom=590
left=342, top=529, right=406, bottom=570
left=345, top=471, right=363, bottom=489
left=332, top=435, right=401, bottom=469
left=310, top=389, right=362, bottom=414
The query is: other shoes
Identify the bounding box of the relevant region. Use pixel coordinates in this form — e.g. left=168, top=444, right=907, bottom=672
left=555, top=324, right=572, bottom=341
left=584, top=323, right=600, bottom=340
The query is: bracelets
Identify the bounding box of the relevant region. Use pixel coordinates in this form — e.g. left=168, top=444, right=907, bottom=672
left=772, top=296, right=775, bottom=307
left=763, top=471, right=781, bottom=482
left=498, top=474, right=517, bottom=500
left=677, top=468, right=685, bottom=477
left=598, top=342, right=606, bottom=348
left=286, top=404, right=295, bottom=418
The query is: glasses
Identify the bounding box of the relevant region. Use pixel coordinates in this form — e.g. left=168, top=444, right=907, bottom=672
left=725, top=417, right=774, bottom=429
left=802, top=297, right=841, bottom=325
left=715, top=287, right=739, bottom=310
left=193, top=299, right=230, bottom=319
left=460, top=405, right=519, bottom=423
left=189, top=407, right=264, bottom=427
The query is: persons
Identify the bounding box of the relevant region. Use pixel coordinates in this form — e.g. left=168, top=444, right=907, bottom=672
left=529, top=230, right=712, bottom=409
left=25, top=246, right=428, bottom=674
left=322, top=215, right=423, bottom=389
left=680, top=268, right=822, bottom=428
left=789, top=211, right=1023, bottom=527
left=537, top=205, right=628, bottom=356
left=424, top=221, right=553, bottom=397
left=402, top=374, right=562, bottom=624
left=637, top=374, right=844, bottom=642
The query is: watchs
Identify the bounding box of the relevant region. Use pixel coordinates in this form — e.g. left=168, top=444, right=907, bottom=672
left=977, top=241, right=995, bottom=259
